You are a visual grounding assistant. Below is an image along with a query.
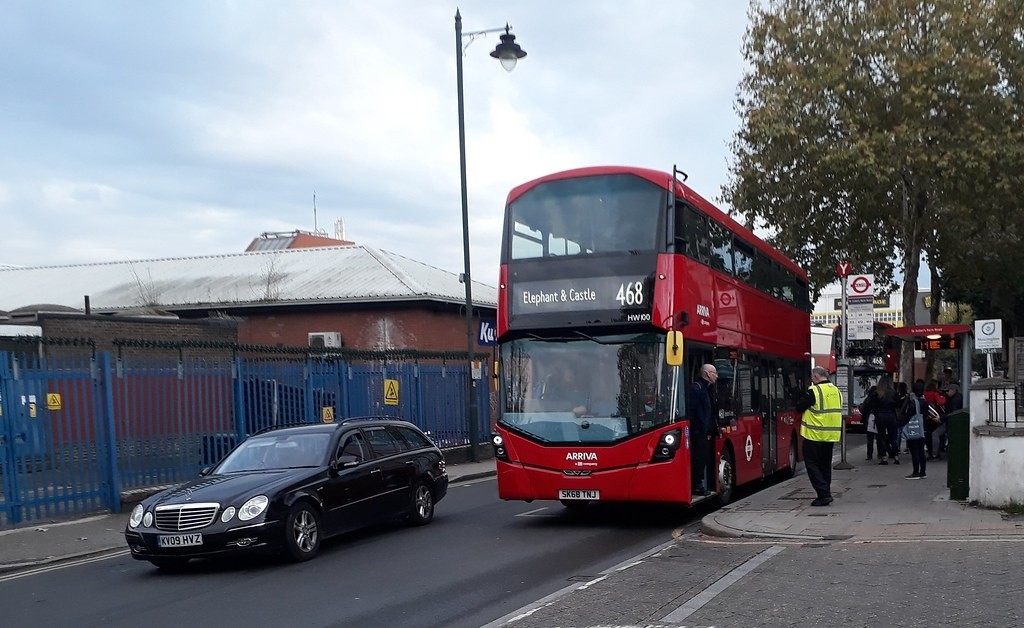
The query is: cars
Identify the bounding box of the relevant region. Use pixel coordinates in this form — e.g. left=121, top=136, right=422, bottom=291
left=122, top=417, right=449, bottom=573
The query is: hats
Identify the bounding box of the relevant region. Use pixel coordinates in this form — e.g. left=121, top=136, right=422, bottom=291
left=949, top=384, right=960, bottom=391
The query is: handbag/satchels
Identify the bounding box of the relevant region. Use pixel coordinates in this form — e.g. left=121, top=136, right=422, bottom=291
left=895, top=395, right=909, bottom=421
left=867, top=413, right=878, bottom=434
left=896, top=396, right=925, bottom=440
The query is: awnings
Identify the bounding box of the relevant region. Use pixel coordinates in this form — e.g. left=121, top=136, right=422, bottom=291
left=882, top=324, right=974, bottom=408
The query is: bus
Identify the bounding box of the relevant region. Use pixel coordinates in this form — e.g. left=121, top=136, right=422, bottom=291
left=488, top=164, right=815, bottom=517
left=825, top=322, right=902, bottom=431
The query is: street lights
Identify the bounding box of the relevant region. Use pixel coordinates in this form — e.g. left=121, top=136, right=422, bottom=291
left=455, top=26, right=528, bottom=460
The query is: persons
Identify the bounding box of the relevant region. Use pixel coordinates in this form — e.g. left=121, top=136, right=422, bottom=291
left=690, top=364, right=720, bottom=496
left=538, top=356, right=588, bottom=418
left=795, top=366, right=843, bottom=506
left=859, top=376, right=963, bottom=480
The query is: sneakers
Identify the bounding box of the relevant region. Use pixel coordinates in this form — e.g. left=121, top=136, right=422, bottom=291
left=905, top=472, right=927, bottom=480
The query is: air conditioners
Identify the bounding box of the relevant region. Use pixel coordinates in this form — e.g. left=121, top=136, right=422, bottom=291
left=307, top=332, right=344, bottom=357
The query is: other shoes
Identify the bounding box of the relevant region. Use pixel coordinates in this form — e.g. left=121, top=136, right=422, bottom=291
left=878, top=461, right=888, bottom=465
left=811, top=495, right=833, bottom=506
left=927, top=455, right=941, bottom=462
left=692, top=487, right=711, bottom=496
left=894, top=459, right=900, bottom=464
left=866, top=458, right=872, bottom=460
left=898, top=450, right=901, bottom=454
left=903, top=449, right=909, bottom=455
